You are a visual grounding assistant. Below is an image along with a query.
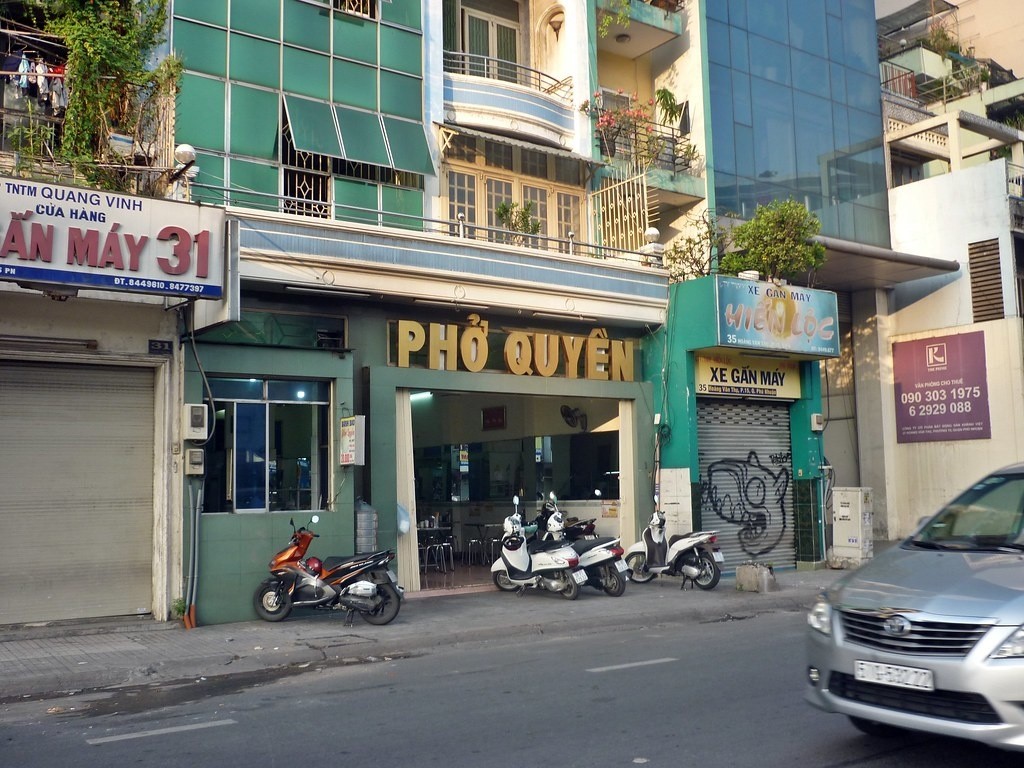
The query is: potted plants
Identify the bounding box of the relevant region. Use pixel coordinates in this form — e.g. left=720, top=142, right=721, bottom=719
left=914, top=24, right=989, bottom=98
left=645, top=88, right=702, bottom=173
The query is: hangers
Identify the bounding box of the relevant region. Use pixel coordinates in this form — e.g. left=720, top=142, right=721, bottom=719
left=4, top=38, right=63, bottom=68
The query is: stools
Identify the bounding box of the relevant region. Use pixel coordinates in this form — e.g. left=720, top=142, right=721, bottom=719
left=419, top=534, right=459, bottom=575
left=461, top=537, right=501, bottom=568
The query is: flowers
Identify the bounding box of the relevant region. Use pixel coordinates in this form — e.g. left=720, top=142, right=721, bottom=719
left=579, top=87, right=654, bottom=138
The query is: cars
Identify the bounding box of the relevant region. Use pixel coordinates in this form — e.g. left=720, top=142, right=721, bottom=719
left=801, top=461, right=1024, bottom=754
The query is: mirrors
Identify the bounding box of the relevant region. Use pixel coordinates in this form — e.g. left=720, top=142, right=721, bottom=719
left=413, top=431, right=620, bottom=502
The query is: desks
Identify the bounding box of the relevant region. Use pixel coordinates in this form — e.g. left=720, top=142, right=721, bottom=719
left=465, top=523, right=503, bottom=564
left=418, top=526, right=452, bottom=574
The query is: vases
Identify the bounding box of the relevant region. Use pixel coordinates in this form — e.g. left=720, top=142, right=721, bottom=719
left=599, top=139, right=616, bottom=156
left=650, top=0, right=678, bottom=13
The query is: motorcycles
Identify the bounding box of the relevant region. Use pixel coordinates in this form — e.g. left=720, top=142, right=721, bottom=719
left=252, top=515, right=406, bottom=627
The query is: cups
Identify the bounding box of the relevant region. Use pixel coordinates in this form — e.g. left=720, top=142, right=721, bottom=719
left=420, top=520, right=429, bottom=528
left=433, top=516, right=440, bottom=529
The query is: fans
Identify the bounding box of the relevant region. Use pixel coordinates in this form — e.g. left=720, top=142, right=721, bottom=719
left=561, top=404, right=587, bottom=431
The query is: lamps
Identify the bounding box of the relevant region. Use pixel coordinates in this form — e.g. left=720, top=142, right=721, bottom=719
left=550, top=20, right=562, bottom=41
left=410, top=391, right=434, bottom=400
left=567, top=232, right=575, bottom=239
left=412, top=299, right=490, bottom=310
left=456, top=212, right=465, bottom=221
left=533, top=312, right=597, bottom=321
left=285, top=286, right=370, bottom=297
left=174, top=144, right=196, bottom=163
left=616, top=34, right=630, bottom=44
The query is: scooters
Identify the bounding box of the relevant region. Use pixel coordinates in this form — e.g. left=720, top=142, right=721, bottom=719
left=620, top=494, right=725, bottom=589
left=490, top=490, right=630, bottom=600
